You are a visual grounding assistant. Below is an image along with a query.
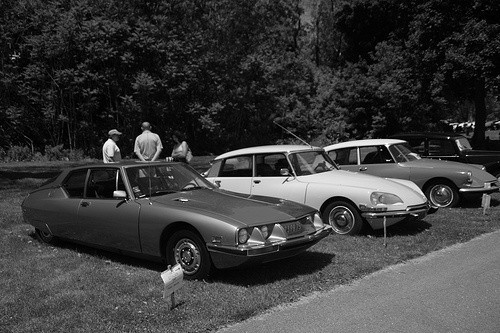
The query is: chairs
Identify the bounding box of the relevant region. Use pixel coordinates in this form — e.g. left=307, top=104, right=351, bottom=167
left=250, top=159, right=288, bottom=176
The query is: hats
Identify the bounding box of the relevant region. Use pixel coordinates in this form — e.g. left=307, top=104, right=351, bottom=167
left=108, top=129, right=122, bottom=135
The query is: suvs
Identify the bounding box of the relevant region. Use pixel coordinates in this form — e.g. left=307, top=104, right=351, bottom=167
left=391, top=133, right=500, bottom=177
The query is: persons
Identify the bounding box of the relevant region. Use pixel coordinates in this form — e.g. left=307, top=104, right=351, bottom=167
left=463, top=124, right=471, bottom=136
left=171, top=130, right=194, bottom=164
left=134, top=122, right=163, bottom=178
left=102, top=129, right=123, bottom=178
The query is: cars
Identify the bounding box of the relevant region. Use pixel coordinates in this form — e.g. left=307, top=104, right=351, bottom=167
left=322, top=139, right=499, bottom=210
left=181, top=144, right=439, bottom=237
left=22, top=161, right=332, bottom=280
left=485, top=121, right=500, bottom=140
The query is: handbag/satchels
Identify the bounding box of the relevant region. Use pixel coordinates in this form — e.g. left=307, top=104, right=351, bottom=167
left=185, top=148, right=193, bottom=165
